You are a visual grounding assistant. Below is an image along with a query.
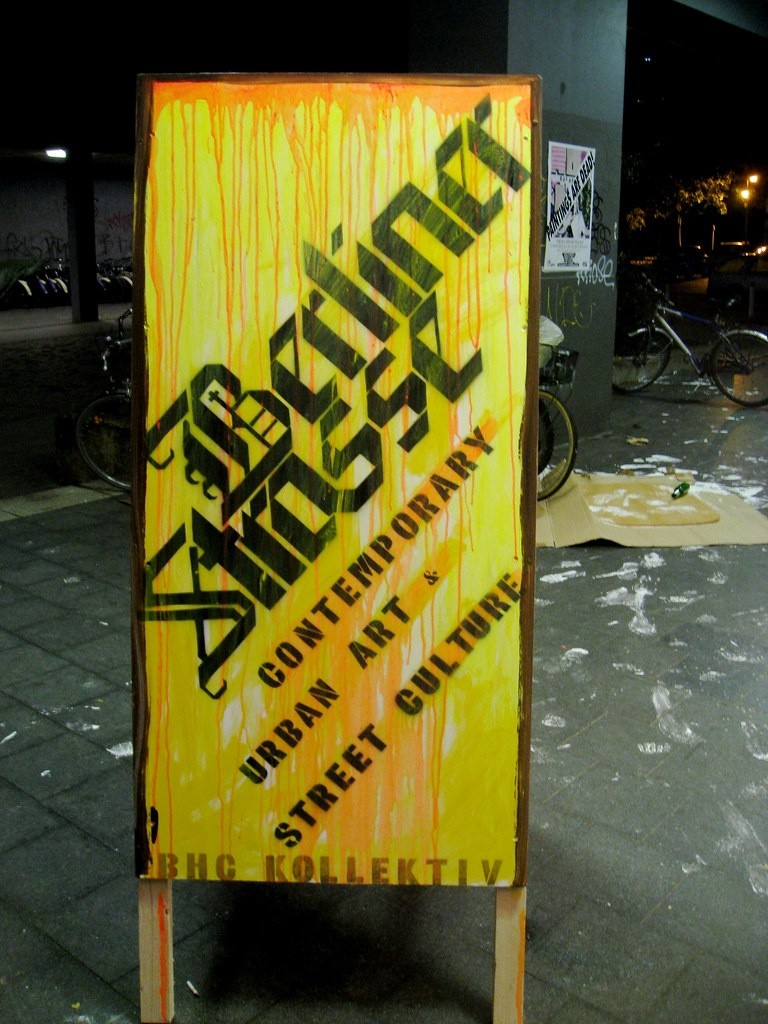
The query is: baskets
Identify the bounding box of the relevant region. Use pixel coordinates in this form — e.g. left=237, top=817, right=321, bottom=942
left=538, top=342, right=583, bottom=386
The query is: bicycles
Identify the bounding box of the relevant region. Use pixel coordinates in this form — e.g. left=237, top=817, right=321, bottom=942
left=0, top=236, right=768, bottom=511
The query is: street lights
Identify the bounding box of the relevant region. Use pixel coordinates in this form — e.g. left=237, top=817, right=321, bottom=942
left=744, top=175, right=758, bottom=249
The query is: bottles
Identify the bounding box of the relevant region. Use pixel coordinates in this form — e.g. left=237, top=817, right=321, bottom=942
left=671, top=481, right=690, bottom=499
